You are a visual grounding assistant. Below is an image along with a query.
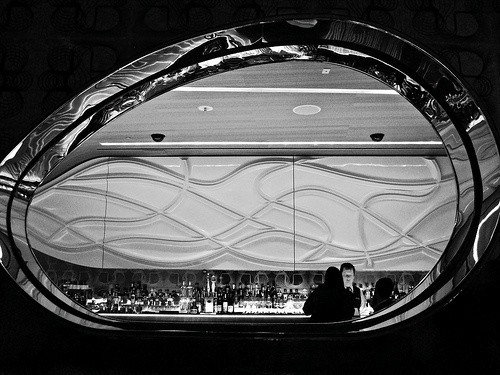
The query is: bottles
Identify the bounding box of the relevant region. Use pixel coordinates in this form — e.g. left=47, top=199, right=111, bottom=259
left=62, top=271, right=423, bottom=317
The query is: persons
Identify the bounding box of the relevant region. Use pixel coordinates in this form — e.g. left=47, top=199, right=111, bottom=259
left=340, top=263, right=368, bottom=318
left=303, top=267, right=355, bottom=323
left=53, top=273, right=424, bottom=315
